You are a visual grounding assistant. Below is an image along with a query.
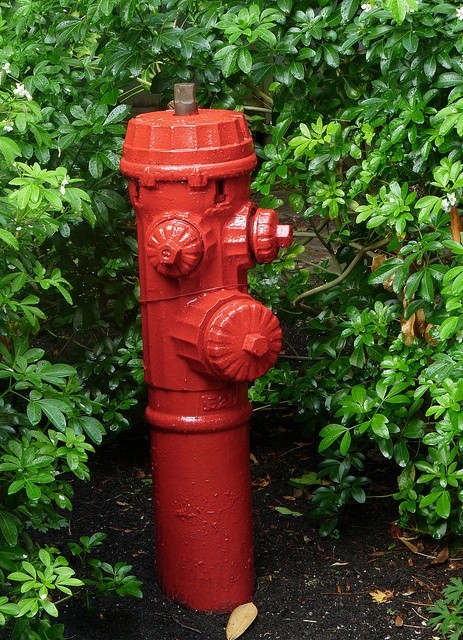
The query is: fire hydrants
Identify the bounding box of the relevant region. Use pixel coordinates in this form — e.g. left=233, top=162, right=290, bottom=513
left=119, top=82, right=293, bottom=614
